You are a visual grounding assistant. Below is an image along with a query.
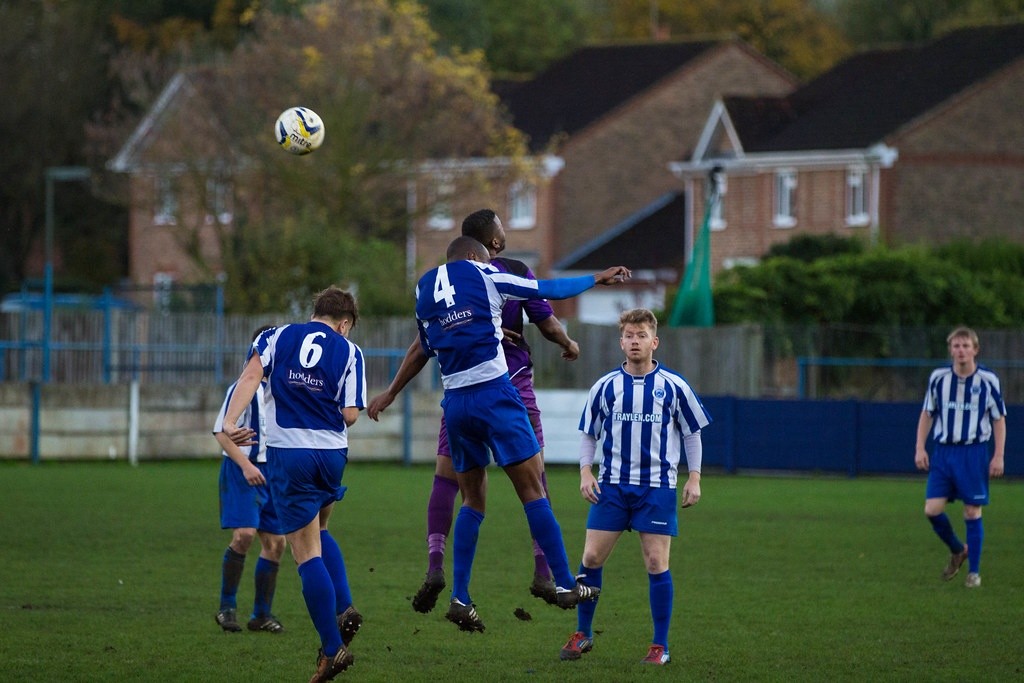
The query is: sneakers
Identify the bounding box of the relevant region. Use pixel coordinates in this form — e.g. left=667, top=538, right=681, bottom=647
left=638, top=644, right=672, bottom=667
left=965, top=572, right=981, bottom=588
left=411, top=571, right=447, bottom=614
left=445, top=595, right=486, bottom=633
left=308, top=643, right=354, bottom=683
left=558, top=630, right=593, bottom=661
left=247, top=616, right=287, bottom=635
left=215, top=608, right=243, bottom=633
left=942, top=543, right=969, bottom=581
left=529, top=575, right=579, bottom=611
left=335, top=607, right=362, bottom=649
left=555, top=574, right=601, bottom=608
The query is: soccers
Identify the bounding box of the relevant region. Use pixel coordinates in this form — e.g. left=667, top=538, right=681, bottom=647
left=274, top=107, right=326, bottom=157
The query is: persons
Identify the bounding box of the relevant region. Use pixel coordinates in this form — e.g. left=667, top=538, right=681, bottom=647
left=414, top=237, right=632, bottom=632
left=222, top=288, right=367, bottom=683
left=367, top=210, right=579, bottom=614
left=213, top=324, right=286, bottom=634
left=915, top=328, right=1007, bottom=586
left=561, top=308, right=711, bottom=665
